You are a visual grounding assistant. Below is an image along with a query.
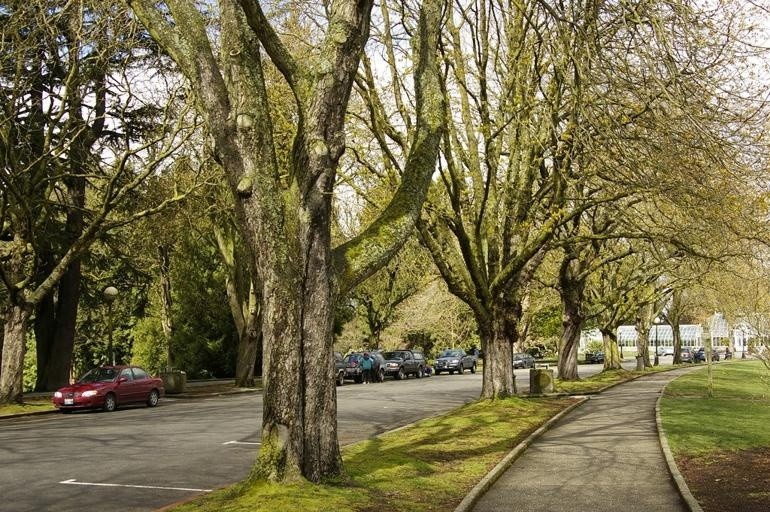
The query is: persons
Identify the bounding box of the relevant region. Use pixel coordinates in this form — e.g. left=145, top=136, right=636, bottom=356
left=359, top=353, right=375, bottom=384
left=424, top=366, right=432, bottom=377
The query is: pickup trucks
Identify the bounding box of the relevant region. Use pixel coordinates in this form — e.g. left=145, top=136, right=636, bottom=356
left=383, top=349, right=425, bottom=380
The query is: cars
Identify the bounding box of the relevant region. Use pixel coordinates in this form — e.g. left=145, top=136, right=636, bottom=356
left=433, top=349, right=478, bottom=374
left=513, top=352, right=536, bottom=369
left=654, top=345, right=746, bottom=363
left=590, top=352, right=605, bottom=363
left=52, top=365, right=165, bottom=414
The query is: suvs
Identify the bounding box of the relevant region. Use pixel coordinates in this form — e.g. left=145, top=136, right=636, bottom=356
left=333, top=350, right=347, bottom=386
left=343, top=348, right=387, bottom=384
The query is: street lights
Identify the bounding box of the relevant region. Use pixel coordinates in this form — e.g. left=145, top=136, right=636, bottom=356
left=103, top=286, right=118, bottom=365
left=618, top=329, right=624, bottom=359
left=741, top=326, right=746, bottom=359
left=653, top=316, right=661, bottom=366
